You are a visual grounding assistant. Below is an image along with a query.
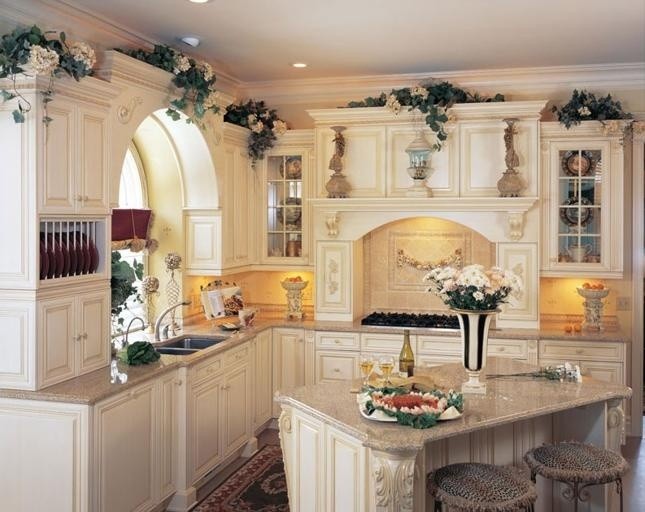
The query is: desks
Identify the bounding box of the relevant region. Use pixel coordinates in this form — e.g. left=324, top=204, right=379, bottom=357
left=275, top=354, right=636, bottom=511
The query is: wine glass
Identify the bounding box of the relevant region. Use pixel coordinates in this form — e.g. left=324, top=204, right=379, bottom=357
left=358, top=355, right=398, bottom=390
left=576, top=286, right=611, bottom=332
left=278, top=277, right=309, bottom=320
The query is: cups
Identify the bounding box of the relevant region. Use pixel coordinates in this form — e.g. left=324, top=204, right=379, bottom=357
left=237, top=310, right=258, bottom=329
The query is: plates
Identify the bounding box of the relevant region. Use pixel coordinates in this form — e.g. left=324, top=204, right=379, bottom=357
left=274, top=197, right=301, bottom=226
left=39, top=232, right=100, bottom=281
left=279, top=157, right=302, bottom=180
left=559, top=195, right=596, bottom=226
left=561, top=151, right=595, bottom=178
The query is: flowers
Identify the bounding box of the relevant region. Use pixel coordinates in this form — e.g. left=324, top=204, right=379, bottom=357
left=116, top=42, right=222, bottom=130
left=421, top=262, right=525, bottom=311
left=339, top=76, right=505, bottom=152
left=552, top=86, right=637, bottom=130
left=366, top=386, right=464, bottom=428
left=0, top=24, right=99, bottom=125
left=224, top=97, right=292, bottom=162
left=486, top=360, right=585, bottom=384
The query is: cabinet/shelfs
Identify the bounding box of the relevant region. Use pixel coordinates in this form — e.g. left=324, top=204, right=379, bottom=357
left=541, top=340, right=628, bottom=415
left=1, top=92, right=109, bottom=216
left=0, top=371, right=179, bottom=512
left=178, top=340, right=257, bottom=498
left=419, top=336, right=538, bottom=370
left=316, top=332, right=416, bottom=388
left=541, top=129, right=625, bottom=278
left=1, top=282, right=109, bottom=394
left=253, top=134, right=310, bottom=265
left=255, top=327, right=306, bottom=437
left=186, top=144, right=252, bottom=275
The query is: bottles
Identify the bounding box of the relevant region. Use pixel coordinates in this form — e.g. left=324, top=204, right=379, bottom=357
left=399, top=330, right=415, bottom=378
left=286, top=239, right=300, bottom=257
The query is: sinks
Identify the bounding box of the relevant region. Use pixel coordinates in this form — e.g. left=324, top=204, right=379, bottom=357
left=158, top=333, right=231, bottom=348
left=150, top=348, right=198, bottom=356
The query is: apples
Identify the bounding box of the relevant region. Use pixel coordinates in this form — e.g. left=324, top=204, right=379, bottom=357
left=564, top=326, right=572, bottom=333
left=574, top=325, right=582, bottom=331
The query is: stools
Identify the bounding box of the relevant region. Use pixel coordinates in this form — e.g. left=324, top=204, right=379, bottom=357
left=521, top=439, right=631, bottom=510
left=426, top=461, right=539, bottom=512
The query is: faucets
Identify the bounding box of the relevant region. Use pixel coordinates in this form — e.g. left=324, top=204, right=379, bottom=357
left=153, top=299, right=193, bottom=341
left=125, top=316, right=146, bottom=347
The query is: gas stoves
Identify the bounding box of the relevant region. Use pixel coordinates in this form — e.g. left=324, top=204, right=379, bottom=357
left=361, top=310, right=460, bottom=329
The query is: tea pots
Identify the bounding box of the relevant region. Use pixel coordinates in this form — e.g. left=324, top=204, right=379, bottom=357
left=565, top=242, right=594, bottom=264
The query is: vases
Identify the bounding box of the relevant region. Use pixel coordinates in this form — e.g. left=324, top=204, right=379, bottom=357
left=450, top=305, right=500, bottom=396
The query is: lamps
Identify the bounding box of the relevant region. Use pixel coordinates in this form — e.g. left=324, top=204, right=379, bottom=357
left=403, top=135, right=433, bottom=179
